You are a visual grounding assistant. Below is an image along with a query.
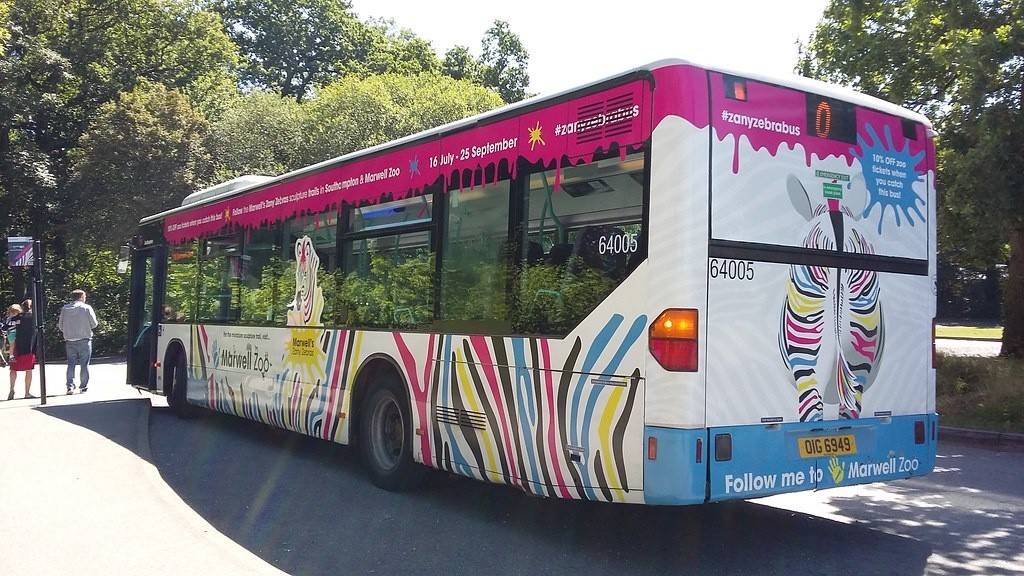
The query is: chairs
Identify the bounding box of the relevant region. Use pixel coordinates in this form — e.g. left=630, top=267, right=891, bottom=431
left=524, top=226, right=642, bottom=297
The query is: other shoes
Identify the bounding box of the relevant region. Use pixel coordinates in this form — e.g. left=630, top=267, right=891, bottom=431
left=8, top=357, right=17, bottom=362
left=67, top=387, right=74, bottom=394
left=80, top=388, right=87, bottom=393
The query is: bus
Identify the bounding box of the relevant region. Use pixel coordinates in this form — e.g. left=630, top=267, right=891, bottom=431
left=117, top=57, right=940, bottom=507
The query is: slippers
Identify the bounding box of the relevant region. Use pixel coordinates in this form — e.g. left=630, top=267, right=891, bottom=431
left=25, top=394, right=36, bottom=399
left=8, top=390, right=15, bottom=400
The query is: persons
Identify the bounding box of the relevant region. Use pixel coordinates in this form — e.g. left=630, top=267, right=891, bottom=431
left=2, top=299, right=36, bottom=400
left=59, top=289, right=98, bottom=396
